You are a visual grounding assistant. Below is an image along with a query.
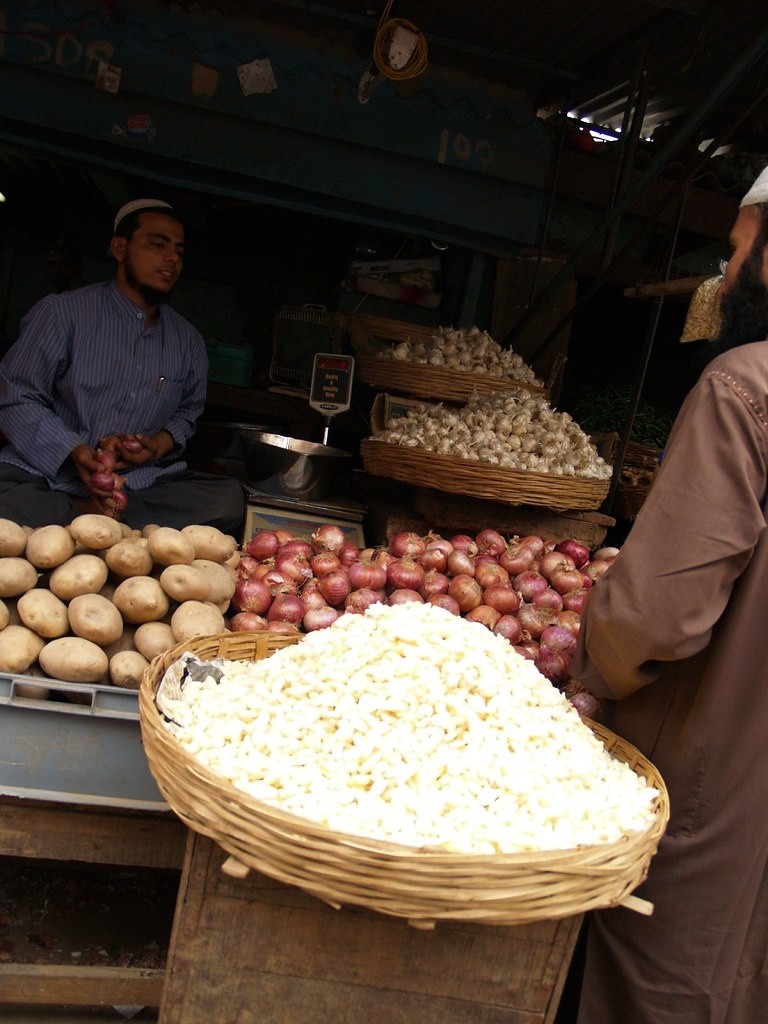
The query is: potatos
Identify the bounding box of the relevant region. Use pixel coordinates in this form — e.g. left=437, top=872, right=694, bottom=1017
left=0, top=515, right=243, bottom=704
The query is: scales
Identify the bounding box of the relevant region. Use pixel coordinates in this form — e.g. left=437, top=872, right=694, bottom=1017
left=242, top=352, right=367, bottom=551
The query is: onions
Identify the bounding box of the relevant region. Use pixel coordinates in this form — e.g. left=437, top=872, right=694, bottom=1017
left=230, top=529, right=620, bottom=720
left=91, top=432, right=144, bottom=515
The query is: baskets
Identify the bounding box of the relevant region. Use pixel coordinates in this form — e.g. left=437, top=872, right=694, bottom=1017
left=358, top=278, right=441, bottom=308
left=138, top=630, right=673, bottom=929
left=351, top=312, right=435, bottom=344
left=356, top=439, right=611, bottom=510
left=352, top=354, right=551, bottom=406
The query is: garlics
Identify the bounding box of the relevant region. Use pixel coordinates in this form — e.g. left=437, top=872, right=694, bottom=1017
left=374, top=389, right=614, bottom=481
left=376, top=324, right=544, bottom=387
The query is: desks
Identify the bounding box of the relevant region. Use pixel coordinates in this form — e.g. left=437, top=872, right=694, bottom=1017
left=201, top=384, right=348, bottom=440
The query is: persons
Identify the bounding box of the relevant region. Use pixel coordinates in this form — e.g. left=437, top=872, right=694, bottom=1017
left=1, top=199, right=247, bottom=543
left=568, top=165, right=768, bottom=1024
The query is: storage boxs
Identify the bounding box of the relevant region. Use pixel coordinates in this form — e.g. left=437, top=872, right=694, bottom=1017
left=202, top=339, right=262, bottom=385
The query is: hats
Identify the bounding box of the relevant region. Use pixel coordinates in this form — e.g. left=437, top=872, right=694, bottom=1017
left=114, top=198, right=172, bottom=232
left=739, top=164, right=768, bottom=210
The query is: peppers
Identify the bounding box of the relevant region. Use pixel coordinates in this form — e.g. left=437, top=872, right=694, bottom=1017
left=557, top=380, right=675, bottom=448
